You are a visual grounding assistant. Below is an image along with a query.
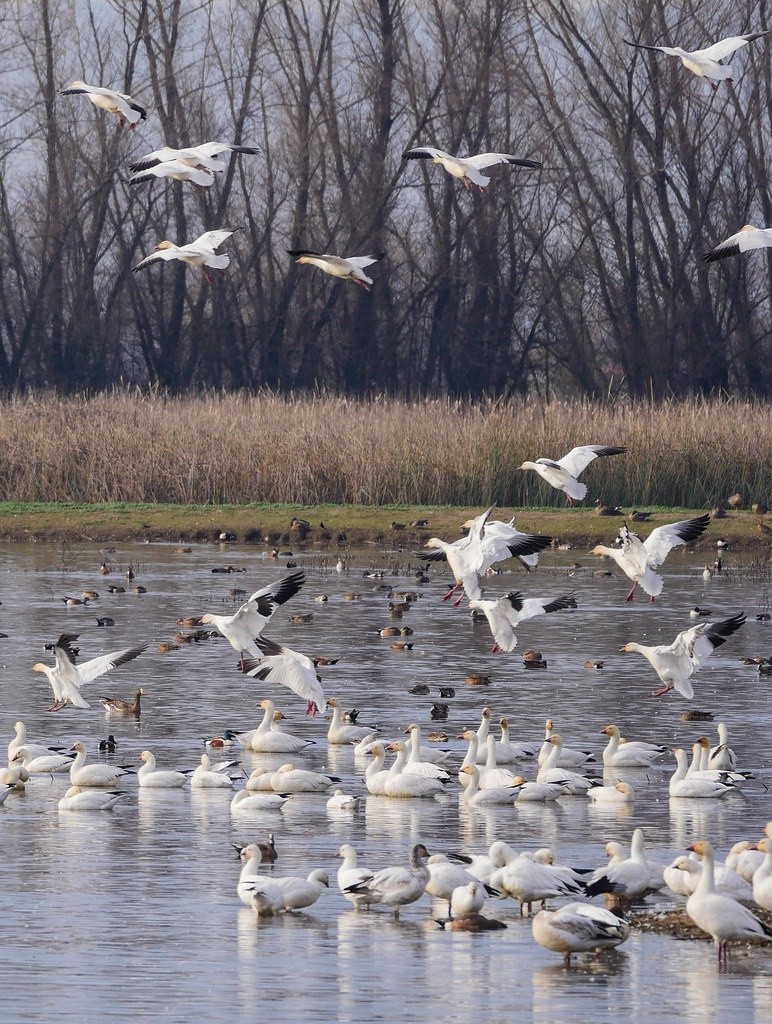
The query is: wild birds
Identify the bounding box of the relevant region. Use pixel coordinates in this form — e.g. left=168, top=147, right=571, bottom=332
left=702, top=225, right=772, bottom=264
left=713, top=494, right=772, bottom=534
left=121, top=161, right=215, bottom=188
left=401, top=147, right=542, bottom=187
left=363, top=519, right=432, bottom=584
left=59, top=547, right=148, bottom=627
left=128, top=142, right=259, bottom=174
left=594, top=499, right=653, bottom=521
left=621, top=29, right=772, bottom=81
left=155, top=572, right=424, bottom=719
left=265, top=519, right=346, bottom=572
left=141, top=524, right=248, bottom=576
left=130, top=227, right=245, bottom=273
left=0, top=633, right=243, bottom=812
left=229, top=694, right=665, bottom=973
left=286, top=249, right=385, bottom=292
left=426, top=500, right=772, bottom=716
left=515, top=445, right=628, bottom=501
left=57, top=80, right=147, bottom=130
left=665, top=720, right=772, bottom=970
